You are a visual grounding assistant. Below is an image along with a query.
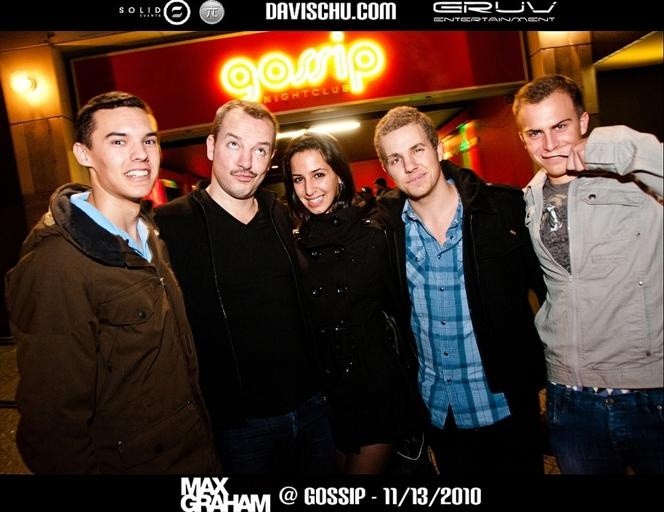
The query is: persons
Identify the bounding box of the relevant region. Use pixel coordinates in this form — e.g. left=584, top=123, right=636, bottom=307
left=148, top=98, right=343, bottom=477
left=361, top=184, right=373, bottom=201
left=7, top=88, right=224, bottom=477
left=511, top=72, right=664, bottom=480
left=374, top=177, right=389, bottom=195
left=282, top=126, right=449, bottom=480
left=360, top=102, right=549, bottom=486
